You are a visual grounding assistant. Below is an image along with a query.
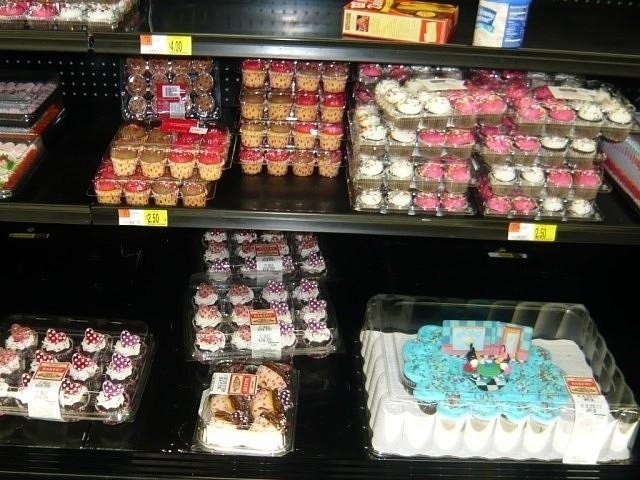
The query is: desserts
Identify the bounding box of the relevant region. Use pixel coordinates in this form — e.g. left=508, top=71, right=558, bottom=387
left=350, top=64, right=632, bottom=218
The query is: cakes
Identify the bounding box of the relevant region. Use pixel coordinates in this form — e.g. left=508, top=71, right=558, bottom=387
left=204, top=229, right=328, bottom=281
left=238, top=60, right=349, bottom=177
left=0, top=323, right=146, bottom=417
left=0, top=0, right=134, bottom=31
left=1, top=138, right=34, bottom=190
left=196, top=357, right=296, bottom=454
left=361, top=318, right=630, bottom=461
left=192, top=277, right=330, bottom=354
left=0, top=79, right=59, bottom=122
left=125, top=57, right=215, bottom=117
left=0, top=106, right=59, bottom=138
left=94, top=122, right=231, bottom=209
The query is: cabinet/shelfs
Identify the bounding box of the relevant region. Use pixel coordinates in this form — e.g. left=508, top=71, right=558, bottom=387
left=84, top=29, right=640, bottom=480
left=0, top=21, right=85, bottom=475
